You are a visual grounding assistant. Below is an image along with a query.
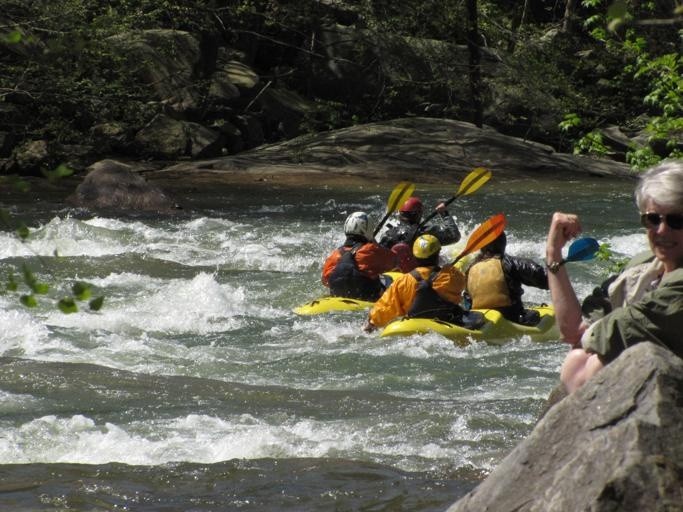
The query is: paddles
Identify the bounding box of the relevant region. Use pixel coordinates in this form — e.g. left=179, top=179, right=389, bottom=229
left=420, top=167, right=491, bottom=227
left=373, top=181, right=415, bottom=237
left=563, top=238, right=600, bottom=263
left=451, top=215, right=505, bottom=267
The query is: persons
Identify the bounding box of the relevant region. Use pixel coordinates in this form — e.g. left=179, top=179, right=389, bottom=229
left=322, top=197, right=549, bottom=334
left=547, top=162, right=683, bottom=395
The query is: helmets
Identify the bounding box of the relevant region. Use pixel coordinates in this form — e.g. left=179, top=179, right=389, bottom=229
left=344, top=211, right=374, bottom=240
left=400, top=197, right=424, bottom=212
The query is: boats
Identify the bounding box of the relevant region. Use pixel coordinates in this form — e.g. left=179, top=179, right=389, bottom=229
left=382, top=303, right=562, bottom=351
left=292, top=251, right=470, bottom=319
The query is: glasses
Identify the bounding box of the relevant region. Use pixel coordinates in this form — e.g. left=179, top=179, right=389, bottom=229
left=641, top=213, right=683, bottom=229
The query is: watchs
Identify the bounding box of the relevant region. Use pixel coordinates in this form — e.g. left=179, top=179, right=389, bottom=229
left=547, top=260, right=564, bottom=273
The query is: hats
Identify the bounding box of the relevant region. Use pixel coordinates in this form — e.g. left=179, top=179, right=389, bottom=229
left=413, top=234, right=441, bottom=259
